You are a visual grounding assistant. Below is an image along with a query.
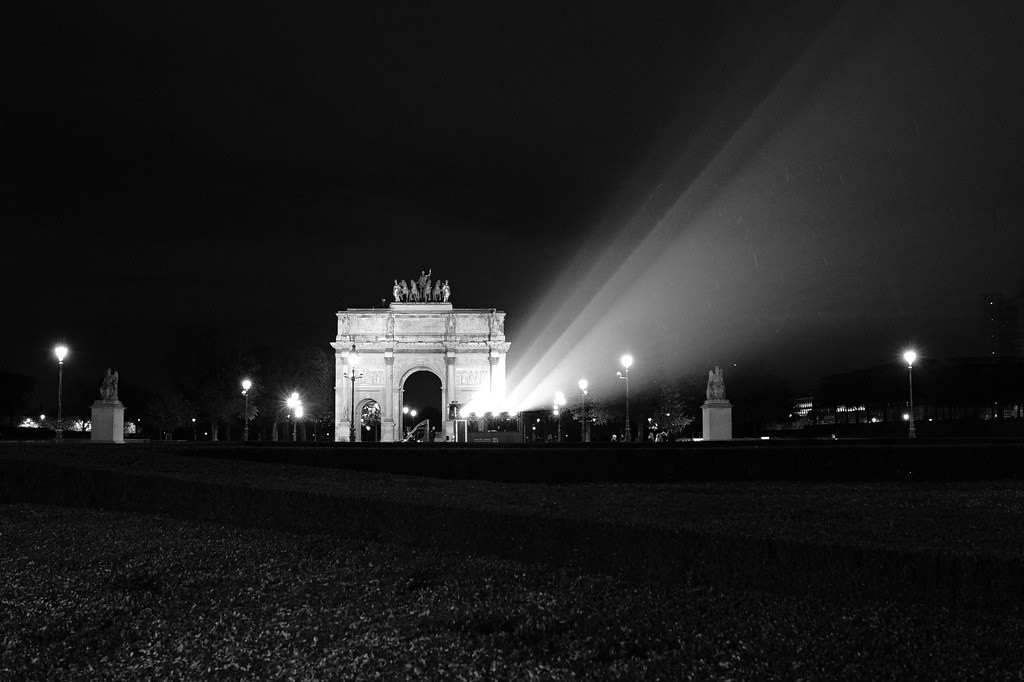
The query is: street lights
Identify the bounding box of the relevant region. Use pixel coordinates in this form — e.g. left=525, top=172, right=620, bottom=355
left=554, top=389, right=566, bottom=442
left=410, top=409, right=416, bottom=430
left=343, top=343, right=363, bottom=443
left=241, top=379, right=252, bottom=441
left=578, top=377, right=588, bottom=443
left=54, top=346, right=69, bottom=442
left=903, top=350, right=917, bottom=439
left=403, top=405, right=409, bottom=440
left=617, top=352, right=634, bottom=442
left=287, top=392, right=303, bottom=441
